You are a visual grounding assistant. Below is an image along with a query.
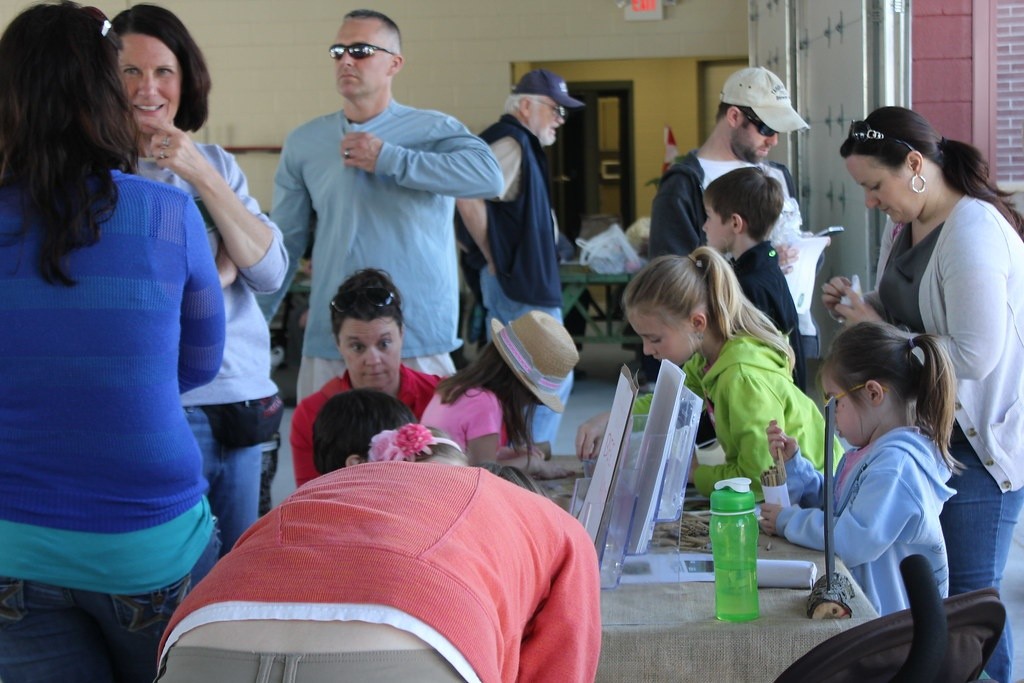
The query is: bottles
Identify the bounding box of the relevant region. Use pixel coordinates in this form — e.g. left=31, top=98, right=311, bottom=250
left=708, top=477, right=760, bottom=622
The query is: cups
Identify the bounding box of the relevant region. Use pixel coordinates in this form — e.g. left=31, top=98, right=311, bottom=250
left=761, top=482, right=791, bottom=508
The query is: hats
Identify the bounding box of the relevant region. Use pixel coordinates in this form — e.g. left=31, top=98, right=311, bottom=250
left=512, top=70, right=586, bottom=108
left=490, top=311, right=580, bottom=414
left=719, top=66, right=808, bottom=133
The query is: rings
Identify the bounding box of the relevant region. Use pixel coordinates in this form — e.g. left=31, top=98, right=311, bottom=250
left=163, top=137, right=168, bottom=145
left=160, top=149, right=164, bottom=159
left=345, top=151, right=349, bottom=158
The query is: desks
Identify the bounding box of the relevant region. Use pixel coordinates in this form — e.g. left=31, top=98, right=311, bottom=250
left=557, top=262, right=645, bottom=345
left=530, top=454, right=878, bottom=683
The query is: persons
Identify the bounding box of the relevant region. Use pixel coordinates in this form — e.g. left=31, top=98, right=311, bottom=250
left=453, top=70, right=585, bottom=455
left=821, top=106, right=1024, bottom=683
left=289, top=268, right=449, bottom=489
left=109, top=4, right=290, bottom=559
left=648, top=66, right=831, bottom=359
left=155, top=462, right=602, bottom=683
left=254, top=8, right=505, bottom=406
left=0, top=0, right=226, bottom=683
left=368, top=423, right=468, bottom=467
left=758, top=320, right=967, bottom=617
left=419, top=310, right=580, bottom=479
left=312, top=388, right=418, bottom=475
left=695, top=167, right=806, bottom=450
left=574, top=246, right=845, bottom=503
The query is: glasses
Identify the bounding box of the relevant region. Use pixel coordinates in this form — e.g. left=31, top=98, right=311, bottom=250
left=329, top=43, right=396, bottom=59
left=736, top=106, right=778, bottom=137
left=848, top=120, right=913, bottom=151
left=537, top=99, right=568, bottom=119
left=329, top=288, right=401, bottom=320
left=81, top=6, right=112, bottom=37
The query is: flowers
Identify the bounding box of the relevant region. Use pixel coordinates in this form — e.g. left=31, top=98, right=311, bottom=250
left=366, top=422, right=439, bottom=462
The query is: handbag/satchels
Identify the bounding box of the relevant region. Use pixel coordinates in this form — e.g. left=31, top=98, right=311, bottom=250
left=199, top=394, right=284, bottom=447
left=575, top=224, right=641, bottom=275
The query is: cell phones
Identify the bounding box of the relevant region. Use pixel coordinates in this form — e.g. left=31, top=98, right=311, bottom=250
left=812, top=225, right=845, bottom=238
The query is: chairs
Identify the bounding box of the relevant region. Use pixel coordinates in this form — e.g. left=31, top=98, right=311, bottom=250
left=774, top=588, right=1007, bottom=683
